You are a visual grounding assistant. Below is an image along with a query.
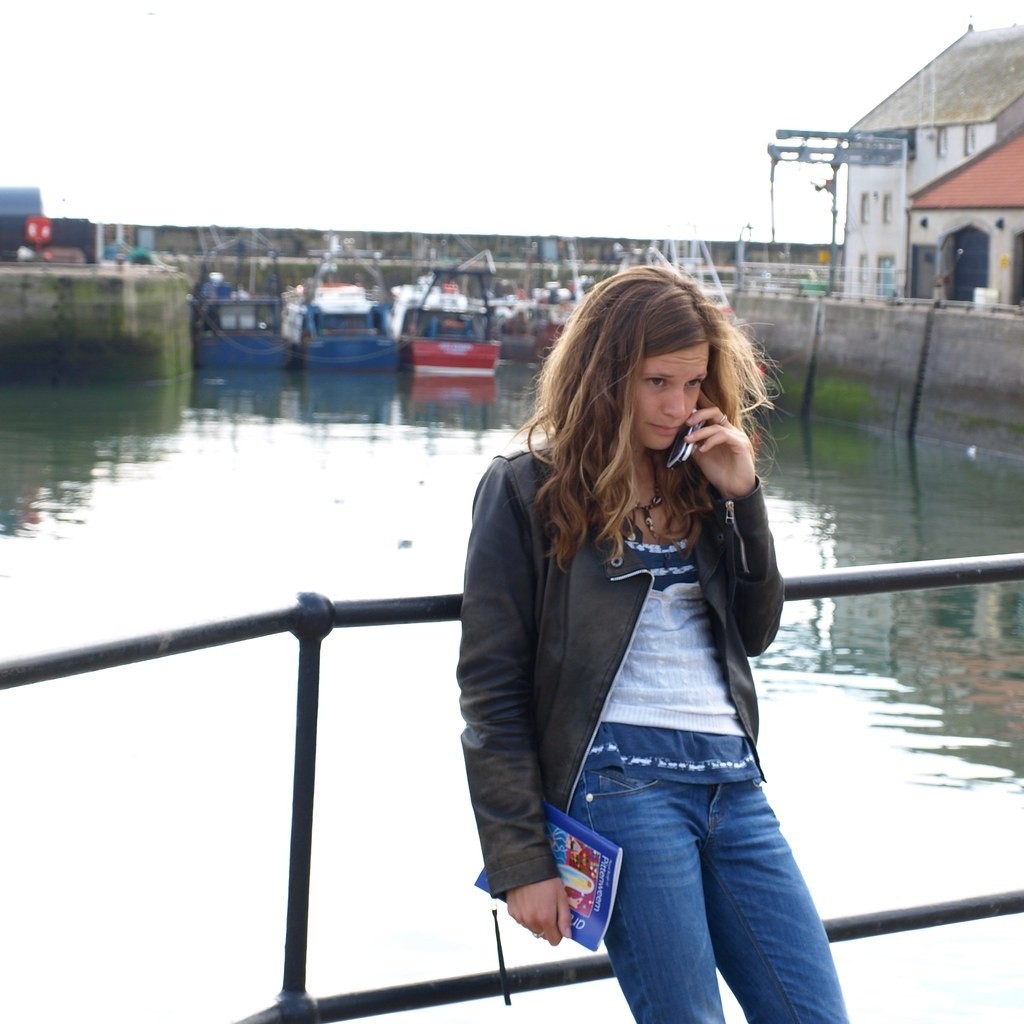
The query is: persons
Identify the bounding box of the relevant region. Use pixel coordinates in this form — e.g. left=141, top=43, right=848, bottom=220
left=455, top=264, right=850, bottom=1023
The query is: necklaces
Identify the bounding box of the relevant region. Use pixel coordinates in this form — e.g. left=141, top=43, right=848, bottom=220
left=633, top=450, right=664, bottom=540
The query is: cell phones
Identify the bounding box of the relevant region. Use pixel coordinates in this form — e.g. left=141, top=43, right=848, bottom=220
left=666, top=402, right=706, bottom=470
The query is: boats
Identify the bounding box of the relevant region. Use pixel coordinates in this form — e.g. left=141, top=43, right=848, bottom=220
left=185, top=225, right=739, bottom=378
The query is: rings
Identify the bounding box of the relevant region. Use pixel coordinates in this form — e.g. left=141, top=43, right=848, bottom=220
left=533, top=931, right=544, bottom=938
left=718, top=413, right=728, bottom=425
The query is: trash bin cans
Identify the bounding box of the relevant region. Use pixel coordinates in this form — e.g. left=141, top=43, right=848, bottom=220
left=973, top=287, right=1000, bottom=312
